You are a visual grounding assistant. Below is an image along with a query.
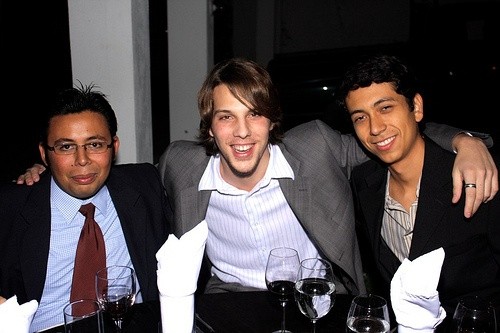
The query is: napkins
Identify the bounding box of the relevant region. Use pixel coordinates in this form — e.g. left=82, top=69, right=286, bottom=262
left=155, top=220, right=208, bottom=333
left=389, top=247, right=446, bottom=333
left=0, top=294, right=38, bottom=333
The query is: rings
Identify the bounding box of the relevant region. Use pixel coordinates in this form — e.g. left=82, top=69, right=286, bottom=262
left=465, top=184, right=476, bottom=188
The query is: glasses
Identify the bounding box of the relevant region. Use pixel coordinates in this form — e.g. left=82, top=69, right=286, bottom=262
left=46, top=141, right=114, bottom=155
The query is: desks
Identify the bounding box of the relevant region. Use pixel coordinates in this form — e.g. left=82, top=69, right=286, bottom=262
left=37, top=291, right=459, bottom=333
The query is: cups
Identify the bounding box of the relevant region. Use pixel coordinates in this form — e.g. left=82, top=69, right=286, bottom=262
left=63, top=299, right=106, bottom=333
left=346, top=294, right=390, bottom=333
left=453, top=297, right=498, bottom=333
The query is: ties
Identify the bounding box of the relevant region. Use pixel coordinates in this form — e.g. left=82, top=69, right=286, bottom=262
left=70, top=202, right=107, bottom=321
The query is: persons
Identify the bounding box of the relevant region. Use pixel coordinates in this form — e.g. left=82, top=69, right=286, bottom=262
left=12, top=59, right=498, bottom=297
left=0, top=88, right=210, bottom=333
left=333, top=52, right=500, bottom=321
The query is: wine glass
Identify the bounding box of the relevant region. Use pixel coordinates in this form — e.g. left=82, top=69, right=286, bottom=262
left=95, top=266, right=135, bottom=329
left=264, top=247, right=302, bottom=333
left=296, top=258, right=336, bottom=333
